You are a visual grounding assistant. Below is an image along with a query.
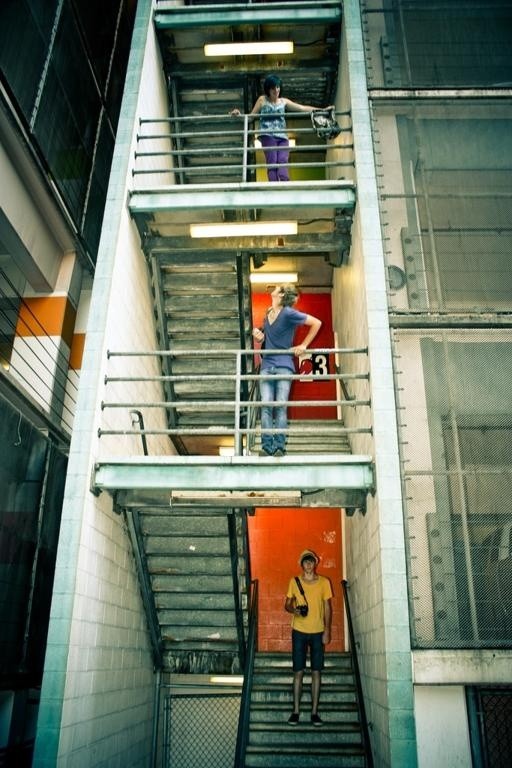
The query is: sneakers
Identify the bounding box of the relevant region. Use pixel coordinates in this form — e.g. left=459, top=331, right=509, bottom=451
left=288, top=710, right=301, bottom=727
left=310, top=711, right=324, bottom=728
left=258, top=447, right=286, bottom=458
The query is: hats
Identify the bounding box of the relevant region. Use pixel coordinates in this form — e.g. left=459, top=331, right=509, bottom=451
left=298, top=549, right=320, bottom=570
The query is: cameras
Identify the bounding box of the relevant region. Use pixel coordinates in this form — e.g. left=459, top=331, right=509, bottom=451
left=296, top=605, right=308, bottom=616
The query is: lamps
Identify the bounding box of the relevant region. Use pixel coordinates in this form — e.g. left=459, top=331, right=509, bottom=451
left=189, top=220, right=298, bottom=238
left=170, top=490, right=301, bottom=508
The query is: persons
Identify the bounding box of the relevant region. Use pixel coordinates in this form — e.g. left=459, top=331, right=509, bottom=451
left=284, top=547, right=334, bottom=727
left=250, top=281, right=323, bottom=457
left=228, top=74, right=337, bottom=181
